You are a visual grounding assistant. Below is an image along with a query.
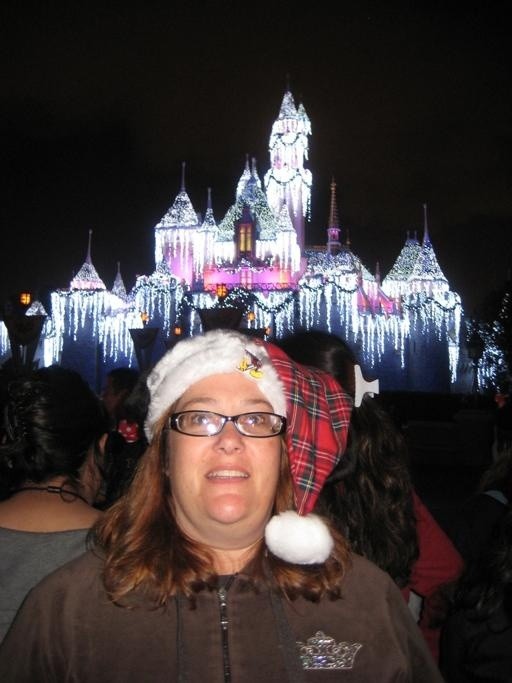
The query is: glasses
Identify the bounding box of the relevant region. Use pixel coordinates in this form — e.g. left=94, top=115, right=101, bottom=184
left=165, top=407, right=289, bottom=441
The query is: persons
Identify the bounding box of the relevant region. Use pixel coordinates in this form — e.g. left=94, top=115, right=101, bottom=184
left=264, top=327, right=510, bottom=683
left=1, top=362, right=121, bottom=651
left=2, top=323, right=446, bottom=683
left=99, top=368, right=138, bottom=406
left=102, top=369, right=155, bottom=516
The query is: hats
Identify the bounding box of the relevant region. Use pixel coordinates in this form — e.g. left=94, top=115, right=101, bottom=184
left=140, top=329, right=357, bottom=566
